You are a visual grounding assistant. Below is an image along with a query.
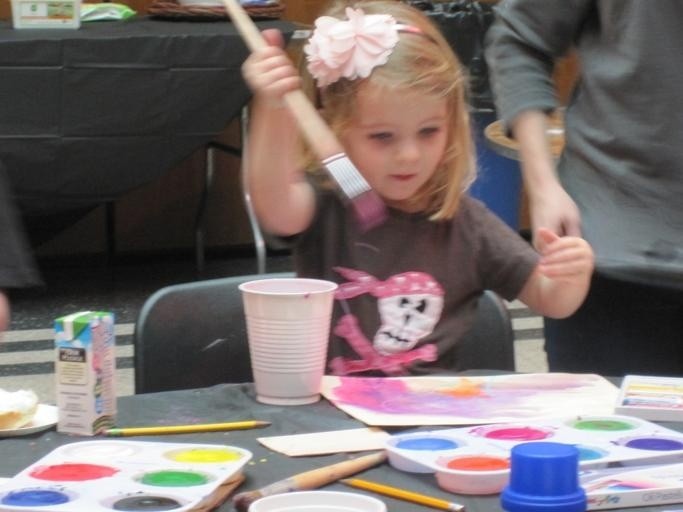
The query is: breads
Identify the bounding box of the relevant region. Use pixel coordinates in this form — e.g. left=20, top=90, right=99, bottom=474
left=0, top=388, right=39, bottom=431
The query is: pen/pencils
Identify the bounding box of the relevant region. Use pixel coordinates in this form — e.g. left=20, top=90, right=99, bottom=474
left=625, top=391, right=683, bottom=399
left=627, top=383, right=682, bottom=391
left=624, top=399, right=682, bottom=408
left=99, top=420, right=272, bottom=436
left=338, top=477, right=465, bottom=512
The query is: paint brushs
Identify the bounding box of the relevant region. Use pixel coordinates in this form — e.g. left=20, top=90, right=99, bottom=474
left=223, top=0, right=390, bottom=235
left=232, top=450, right=390, bottom=511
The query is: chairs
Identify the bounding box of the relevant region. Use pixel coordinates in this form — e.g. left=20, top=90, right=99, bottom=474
left=134, top=272, right=515, bottom=395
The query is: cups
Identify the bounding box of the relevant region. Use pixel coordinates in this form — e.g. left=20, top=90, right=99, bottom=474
left=246, top=490, right=386, bottom=512
left=238, top=277, right=338, bottom=405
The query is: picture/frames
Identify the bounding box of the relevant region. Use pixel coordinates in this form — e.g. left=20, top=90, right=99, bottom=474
left=10, top=0, right=80, bottom=30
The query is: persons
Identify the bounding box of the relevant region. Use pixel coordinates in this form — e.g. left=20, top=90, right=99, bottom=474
left=0, top=181, right=46, bottom=332
left=481, top=0, right=683, bottom=377
left=240, top=0, right=594, bottom=376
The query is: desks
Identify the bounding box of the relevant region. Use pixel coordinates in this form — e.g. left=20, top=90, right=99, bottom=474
left=0, top=13, right=296, bottom=281
left=0, top=376, right=683, bottom=512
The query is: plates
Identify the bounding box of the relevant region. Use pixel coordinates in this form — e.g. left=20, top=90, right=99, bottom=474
left=1, top=403, right=59, bottom=438
